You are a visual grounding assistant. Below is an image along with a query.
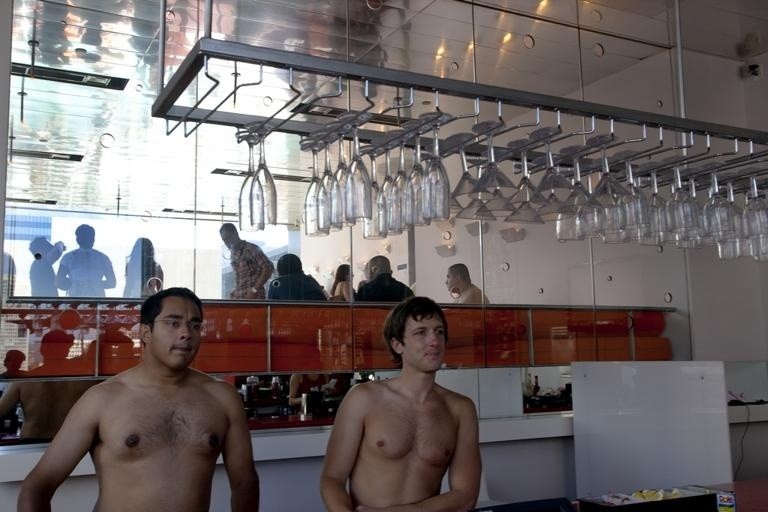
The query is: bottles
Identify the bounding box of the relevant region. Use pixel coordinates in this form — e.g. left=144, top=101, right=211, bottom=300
left=525, top=373, right=540, bottom=396
left=245, top=377, right=260, bottom=408
left=13, top=405, right=24, bottom=437
left=271, top=377, right=281, bottom=399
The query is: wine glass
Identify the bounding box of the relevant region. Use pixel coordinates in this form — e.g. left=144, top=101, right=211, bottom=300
left=556, top=150, right=766, bottom=258
left=446, top=121, right=630, bottom=226
left=303, top=114, right=451, bottom=240
left=235, top=121, right=277, bottom=231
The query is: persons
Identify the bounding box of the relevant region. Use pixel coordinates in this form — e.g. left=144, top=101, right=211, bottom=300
left=17, top=287, right=260, bottom=512
left=320, top=297, right=481, bottom=512
left=0, top=330, right=133, bottom=376
left=0, top=380, right=102, bottom=439
left=288, top=373, right=354, bottom=415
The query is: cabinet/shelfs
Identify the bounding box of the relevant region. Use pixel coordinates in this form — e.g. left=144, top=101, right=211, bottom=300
left=13, top=405, right=24, bottom=437
left=245, top=377, right=260, bottom=408
left=525, top=373, right=540, bottom=396
left=271, top=377, right=281, bottom=399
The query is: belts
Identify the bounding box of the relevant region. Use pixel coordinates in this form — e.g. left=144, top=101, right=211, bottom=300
left=235, top=121, right=277, bottom=231
